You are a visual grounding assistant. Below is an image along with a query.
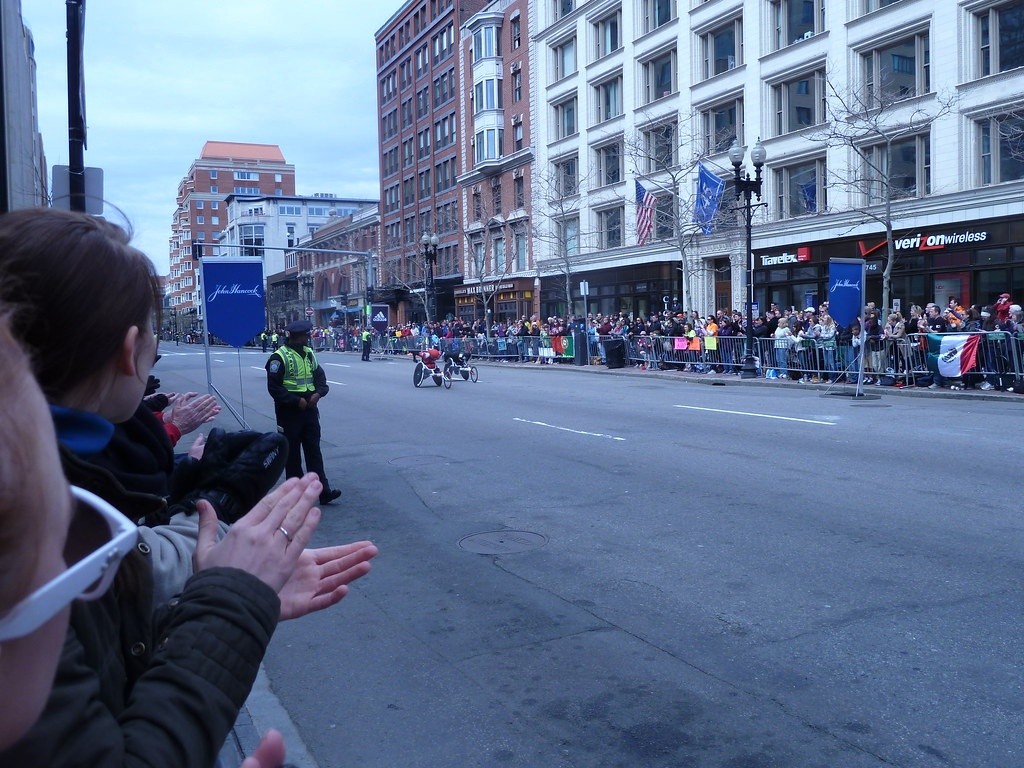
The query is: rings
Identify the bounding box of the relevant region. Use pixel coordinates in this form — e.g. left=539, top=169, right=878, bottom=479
left=277, top=526, right=292, bottom=542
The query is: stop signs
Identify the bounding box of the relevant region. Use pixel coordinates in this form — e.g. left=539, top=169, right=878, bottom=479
left=305, top=308, right=314, bottom=317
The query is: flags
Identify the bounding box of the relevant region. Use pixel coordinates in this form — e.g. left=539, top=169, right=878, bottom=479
left=636, top=180, right=659, bottom=247
left=691, top=160, right=726, bottom=235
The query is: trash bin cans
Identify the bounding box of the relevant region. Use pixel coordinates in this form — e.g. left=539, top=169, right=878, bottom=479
left=603, top=338, right=625, bottom=369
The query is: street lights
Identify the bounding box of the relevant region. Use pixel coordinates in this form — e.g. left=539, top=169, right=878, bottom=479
left=728, top=137, right=768, bottom=378
left=422, top=229, right=438, bottom=327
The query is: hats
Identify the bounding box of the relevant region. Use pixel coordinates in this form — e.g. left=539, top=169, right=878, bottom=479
left=804, top=307, right=815, bottom=312
left=284, top=320, right=313, bottom=336
left=1000, top=293, right=1010, bottom=298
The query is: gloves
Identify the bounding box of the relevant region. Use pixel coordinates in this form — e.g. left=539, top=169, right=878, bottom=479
left=200, top=432, right=290, bottom=526
left=195, top=427, right=264, bottom=496
left=144, top=375, right=161, bottom=396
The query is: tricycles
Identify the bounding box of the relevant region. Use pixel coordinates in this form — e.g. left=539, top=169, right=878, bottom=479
left=413, top=347, right=479, bottom=387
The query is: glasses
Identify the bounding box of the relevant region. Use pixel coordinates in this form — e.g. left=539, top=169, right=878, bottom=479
left=961, top=314, right=966, bottom=316
left=820, top=311, right=824, bottom=313
left=0, top=486, right=139, bottom=640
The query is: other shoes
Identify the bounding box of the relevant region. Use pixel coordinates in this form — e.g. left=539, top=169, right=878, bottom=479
left=320, top=489, right=341, bottom=505
left=676, top=365, right=1014, bottom=392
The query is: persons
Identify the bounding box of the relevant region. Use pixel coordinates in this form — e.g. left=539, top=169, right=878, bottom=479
left=0, top=304, right=286, bottom=768
left=264, top=320, right=342, bottom=505
left=0, top=207, right=379, bottom=768
left=101, top=391, right=291, bottom=618
left=141, top=292, right=1024, bottom=397
